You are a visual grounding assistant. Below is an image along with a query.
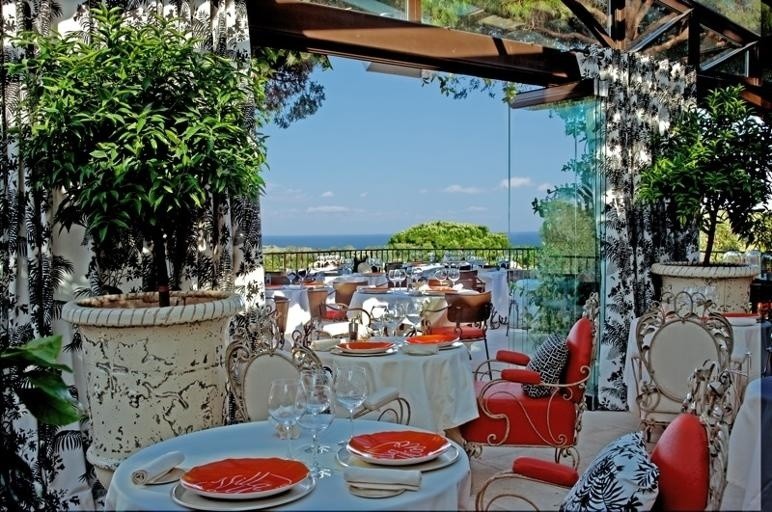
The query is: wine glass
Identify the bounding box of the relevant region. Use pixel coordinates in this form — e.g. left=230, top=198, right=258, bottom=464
left=444, top=254, right=510, bottom=271
left=309, top=300, right=462, bottom=356
left=267, top=366, right=369, bottom=480
left=335, top=258, right=382, bottom=277
left=387, top=266, right=463, bottom=297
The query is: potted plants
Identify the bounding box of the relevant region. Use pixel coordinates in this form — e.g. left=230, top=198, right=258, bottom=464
left=3, top=0, right=279, bottom=472
left=635, top=82, right=772, bottom=321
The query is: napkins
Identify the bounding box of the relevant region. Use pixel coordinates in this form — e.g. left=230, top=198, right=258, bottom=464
left=131, top=451, right=185, bottom=484
left=344, top=466, right=423, bottom=490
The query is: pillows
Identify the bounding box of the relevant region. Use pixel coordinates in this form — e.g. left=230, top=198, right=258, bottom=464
left=520, top=330, right=570, bottom=399
left=558, top=431, right=659, bottom=512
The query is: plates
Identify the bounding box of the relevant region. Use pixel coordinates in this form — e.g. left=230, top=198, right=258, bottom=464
left=148, top=467, right=186, bottom=486
left=171, top=458, right=316, bottom=511
left=336, top=430, right=460, bottom=473
left=362, top=288, right=392, bottom=294
left=349, top=484, right=404, bottom=499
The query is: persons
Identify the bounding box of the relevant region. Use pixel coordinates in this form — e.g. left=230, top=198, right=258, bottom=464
left=371, top=266, right=380, bottom=274
left=458, top=258, right=469, bottom=267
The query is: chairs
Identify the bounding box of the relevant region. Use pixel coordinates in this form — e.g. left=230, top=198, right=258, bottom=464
left=475, top=360, right=751, bottom=512
left=224, top=303, right=412, bottom=426
left=419, top=291, right=493, bottom=381
left=263, top=255, right=575, bottom=357
left=461, top=314, right=593, bottom=469
left=631, top=290, right=749, bottom=443
